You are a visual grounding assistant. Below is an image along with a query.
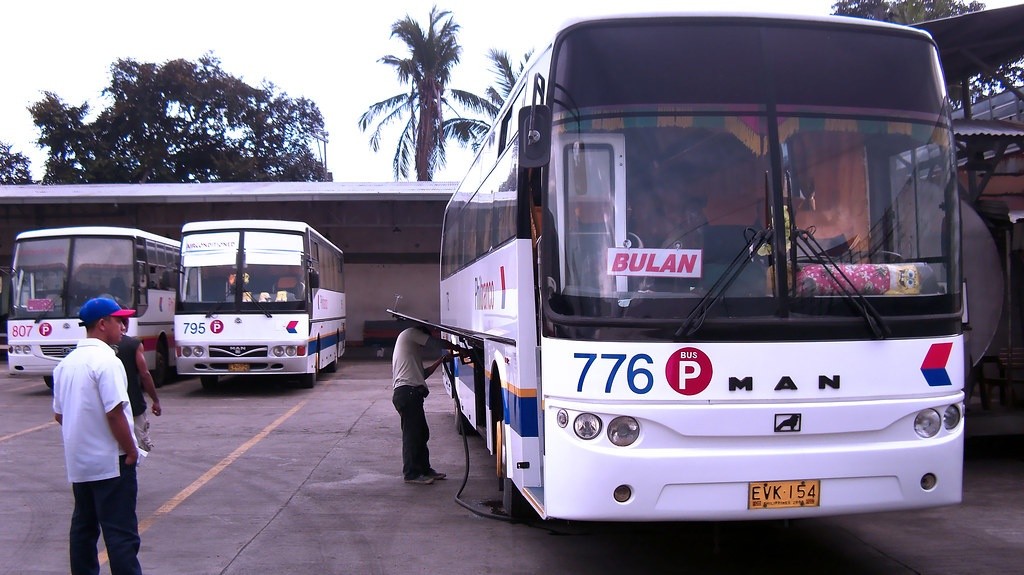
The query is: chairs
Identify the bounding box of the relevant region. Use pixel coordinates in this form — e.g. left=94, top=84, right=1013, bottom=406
left=271, top=273, right=304, bottom=297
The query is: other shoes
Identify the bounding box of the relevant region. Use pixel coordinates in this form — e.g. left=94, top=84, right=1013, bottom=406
left=423, top=467, right=446, bottom=480
left=404, top=475, right=434, bottom=484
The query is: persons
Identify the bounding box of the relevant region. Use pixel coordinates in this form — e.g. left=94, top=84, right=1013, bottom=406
left=110, top=304, right=162, bottom=452
left=392, top=324, right=469, bottom=484
left=53, top=298, right=148, bottom=575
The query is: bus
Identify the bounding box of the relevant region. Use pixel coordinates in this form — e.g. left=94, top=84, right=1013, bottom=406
left=172, top=219, right=346, bottom=389
left=387, top=11, right=986, bottom=527
left=1, top=226, right=201, bottom=392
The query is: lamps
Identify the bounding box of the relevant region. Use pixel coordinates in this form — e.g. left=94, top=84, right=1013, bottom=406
left=392, top=227, right=402, bottom=233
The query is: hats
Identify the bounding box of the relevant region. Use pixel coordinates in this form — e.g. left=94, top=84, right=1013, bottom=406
left=78, top=297, right=136, bottom=327
